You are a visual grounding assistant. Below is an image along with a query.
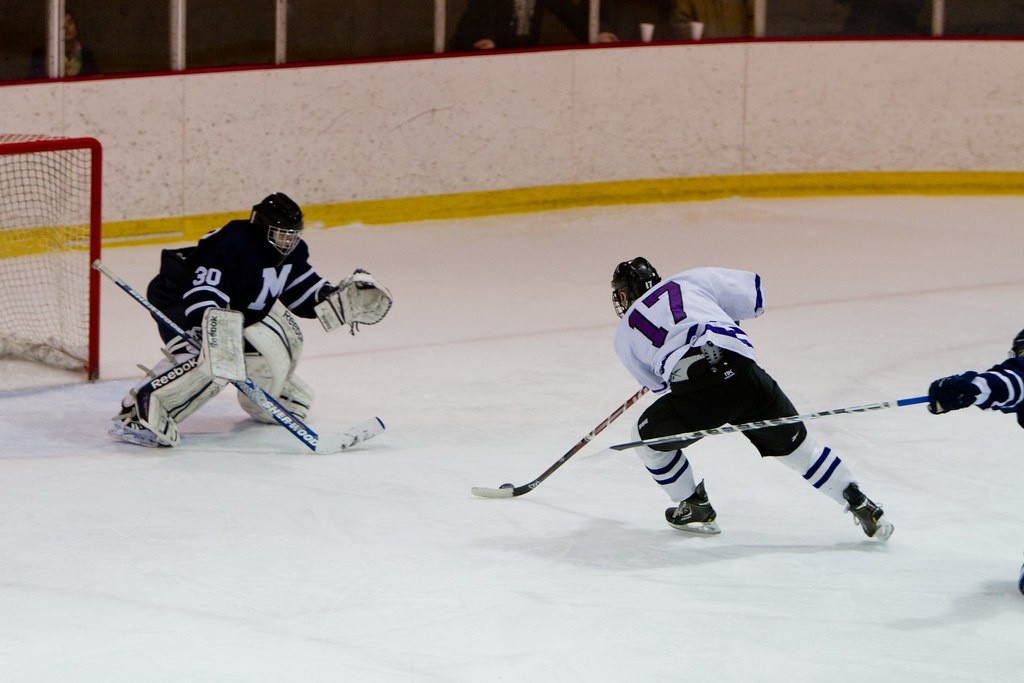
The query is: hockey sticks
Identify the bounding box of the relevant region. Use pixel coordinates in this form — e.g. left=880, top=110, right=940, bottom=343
left=92, top=259, right=385, bottom=455
left=609, top=394, right=931, bottom=451
left=470, top=387, right=650, bottom=499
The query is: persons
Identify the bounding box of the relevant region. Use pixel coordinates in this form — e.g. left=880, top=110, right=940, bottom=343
left=927, top=328, right=1024, bottom=594
left=109, top=192, right=392, bottom=447
left=611, top=257, right=896, bottom=542
left=451, top=0, right=618, bottom=52
left=32, top=10, right=98, bottom=80
left=670, top=0, right=755, bottom=39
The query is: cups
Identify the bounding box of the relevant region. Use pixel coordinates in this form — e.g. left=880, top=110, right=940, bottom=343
left=639, top=22, right=654, bottom=43
left=689, top=21, right=705, bottom=41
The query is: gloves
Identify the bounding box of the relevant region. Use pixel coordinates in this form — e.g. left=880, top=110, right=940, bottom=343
left=928, top=371, right=976, bottom=414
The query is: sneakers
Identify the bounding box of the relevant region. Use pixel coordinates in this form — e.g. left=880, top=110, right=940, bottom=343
left=843, top=482, right=894, bottom=543
left=665, top=478, right=721, bottom=534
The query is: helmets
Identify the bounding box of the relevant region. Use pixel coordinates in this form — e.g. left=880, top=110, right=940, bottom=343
left=249, top=193, right=304, bottom=267
left=611, top=256, right=661, bottom=319
left=1007, top=329, right=1024, bottom=359
